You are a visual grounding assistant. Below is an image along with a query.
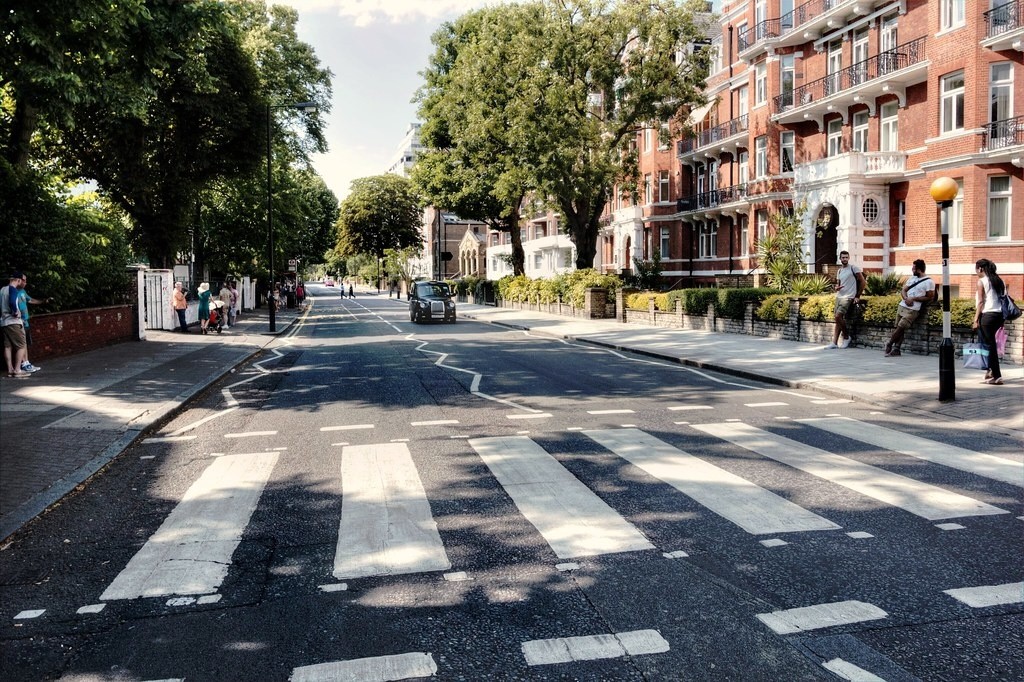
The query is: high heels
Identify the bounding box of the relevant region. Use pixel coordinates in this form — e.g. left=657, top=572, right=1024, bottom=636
left=200, top=327, right=204, bottom=334
left=203, top=328, right=207, bottom=335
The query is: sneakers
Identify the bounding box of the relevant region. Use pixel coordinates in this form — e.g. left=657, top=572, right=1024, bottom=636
left=841, top=336, right=852, bottom=348
left=824, top=342, right=838, bottom=350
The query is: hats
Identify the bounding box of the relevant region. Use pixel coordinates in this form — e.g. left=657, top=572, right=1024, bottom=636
left=8, top=270, right=26, bottom=282
left=197, top=283, right=210, bottom=293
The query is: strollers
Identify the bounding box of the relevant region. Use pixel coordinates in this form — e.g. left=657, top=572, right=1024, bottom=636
left=207, top=304, right=224, bottom=334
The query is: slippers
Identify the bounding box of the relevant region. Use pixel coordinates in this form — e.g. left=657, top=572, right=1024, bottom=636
left=8, top=373, right=13, bottom=378
left=14, top=370, right=31, bottom=377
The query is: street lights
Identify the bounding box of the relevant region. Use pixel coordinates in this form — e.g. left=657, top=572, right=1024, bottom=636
left=373, top=232, right=380, bottom=293
left=930, top=176, right=959, bottom=400
left=266, top=102, right=317, bottom=332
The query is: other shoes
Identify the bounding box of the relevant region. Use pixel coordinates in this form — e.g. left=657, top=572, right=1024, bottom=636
left=885, top=349, right=901, bottom=357
left=884, top=339, right=892, bottom=353
left=221, top=325, right=229, bottom=329
left=21, top=364, right=36, bottom=373
left=183, top=329, right=191, bottom=333
left=29, top=364, right=41, bottom=371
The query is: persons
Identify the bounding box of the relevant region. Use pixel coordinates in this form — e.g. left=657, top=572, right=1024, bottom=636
left=210, top=283, right=240, bottom=328
left=0, top=272, right=31, bottom=377
left=340, top=283, right=347, bottom=299
left=296, top=284, right=303, bottom=309
left=266, top=283, right=287, bottom=312
left=349, top=284, right=355, bottom=299
left=17, top=274, right=45, bottom=372
left=884, top=259, right=935, bottom=357
left=198, top=282, right=217, bottom=334
left=824, top=251, right=866, bottom=349
left=973, top=258, right=1007, bottom=384
left=173, top=282, right=191, bottom=332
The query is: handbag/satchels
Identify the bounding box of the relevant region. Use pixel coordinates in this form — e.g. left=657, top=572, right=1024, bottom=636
left=229, top=308, right=236, bottom=317
left=962, top=328, right=989, bottom=370
left=926, top=288, right=939, bottom=302
left=995, top=326, right=1007, bottom=358
left=1002, top=294, right=1022, bottom=320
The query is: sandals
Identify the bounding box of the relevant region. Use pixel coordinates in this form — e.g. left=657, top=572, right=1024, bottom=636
left=985, top=371, right=992, bottom=379
left=985, top=378, right=1003, bottom=385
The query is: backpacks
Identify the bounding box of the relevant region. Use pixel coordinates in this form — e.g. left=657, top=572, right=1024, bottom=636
left=851, top=265, right=867, bottom=296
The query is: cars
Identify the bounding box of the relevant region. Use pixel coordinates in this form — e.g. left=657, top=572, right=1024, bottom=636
left=325, top=279, right=335, bottom=287
left=407, top=280, right=457, bottom=323
